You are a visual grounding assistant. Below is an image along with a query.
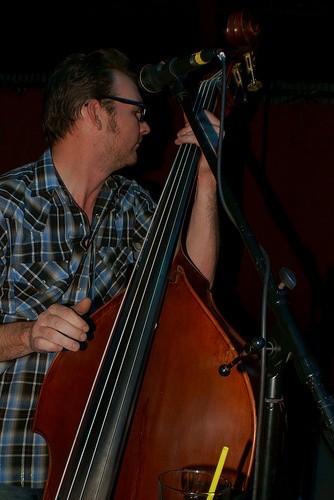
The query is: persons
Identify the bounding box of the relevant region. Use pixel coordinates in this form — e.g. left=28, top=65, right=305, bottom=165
left=2, top=50, right=223, bottom=500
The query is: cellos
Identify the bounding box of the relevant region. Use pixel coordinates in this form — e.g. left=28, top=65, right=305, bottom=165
left=32, top=10, right=261, bottom=500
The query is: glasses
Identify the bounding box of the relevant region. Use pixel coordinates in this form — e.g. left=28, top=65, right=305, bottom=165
left=84, top=94, right=147, bottom=123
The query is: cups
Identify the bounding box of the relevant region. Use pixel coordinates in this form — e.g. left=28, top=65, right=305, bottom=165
left=158, top=469, right=231, bottom=500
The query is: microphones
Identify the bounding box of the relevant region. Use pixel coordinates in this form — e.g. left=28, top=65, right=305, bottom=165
left=137, top=47, right=222, bottom=94
